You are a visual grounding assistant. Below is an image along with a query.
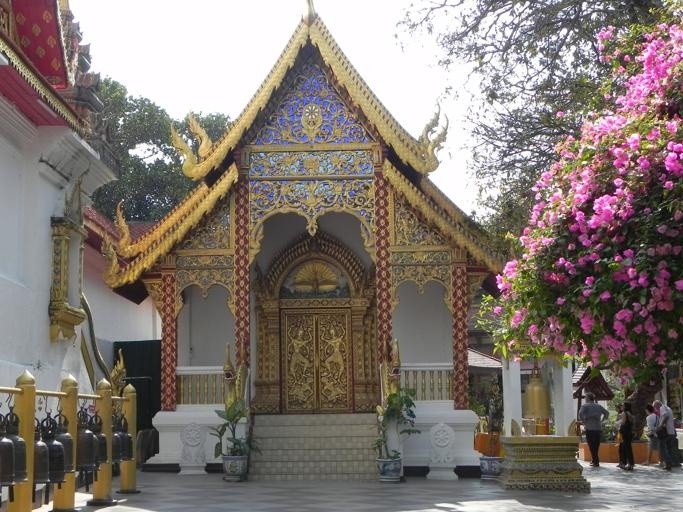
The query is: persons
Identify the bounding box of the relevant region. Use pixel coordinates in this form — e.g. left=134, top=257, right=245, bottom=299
left=651, top=399, right=675, bottom=471
left=640, top=404, right=660, bottom=466
left=618, top=402, right=636, bottom=470
left=578, top=392, right=609, bottom=467
left=319, top=323, right=345, bottom=385
left=614, top=402, right=627, bottom=468
left=286, top=325, right=313, bottom=381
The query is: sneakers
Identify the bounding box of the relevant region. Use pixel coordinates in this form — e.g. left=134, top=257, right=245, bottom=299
left=590, top=461, right=599, bottom=466
left=617, top=463, right=633, bottom=470
left=644, top=460, right=681, bottom=469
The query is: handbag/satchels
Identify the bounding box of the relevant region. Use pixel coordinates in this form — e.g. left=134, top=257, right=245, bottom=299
left=657, top=427, right=667, bottom=439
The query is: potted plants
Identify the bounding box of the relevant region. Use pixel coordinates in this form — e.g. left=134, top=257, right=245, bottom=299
left=207, top=398, right=263, bottom=483
left=367, top=388, right=421, bottom=482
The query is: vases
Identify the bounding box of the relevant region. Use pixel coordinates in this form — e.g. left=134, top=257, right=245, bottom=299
left=480, top=456, right=504, bottom=482
